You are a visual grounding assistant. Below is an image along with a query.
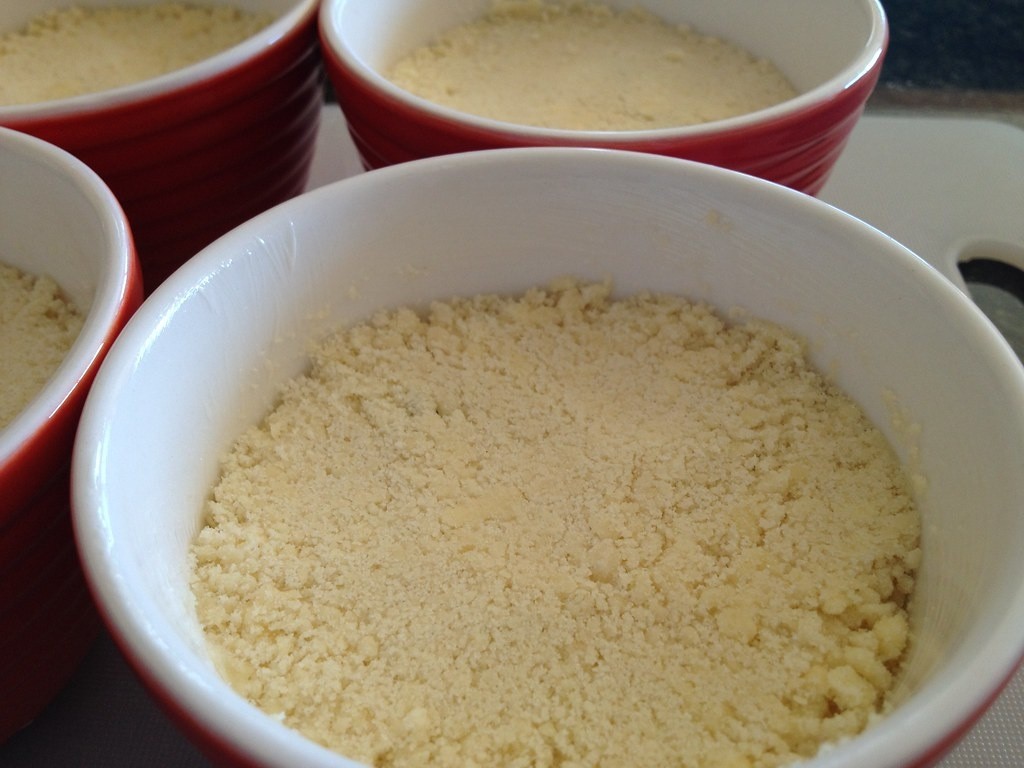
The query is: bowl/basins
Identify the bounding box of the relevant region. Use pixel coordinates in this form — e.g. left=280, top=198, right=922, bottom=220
left=0, top=123, right=146, bottom=740
left=0, top=0, right=319, bottom=295
left=321, top=0, right=890, bottom=200
left=67, top=144, right=1024, bottom=768
